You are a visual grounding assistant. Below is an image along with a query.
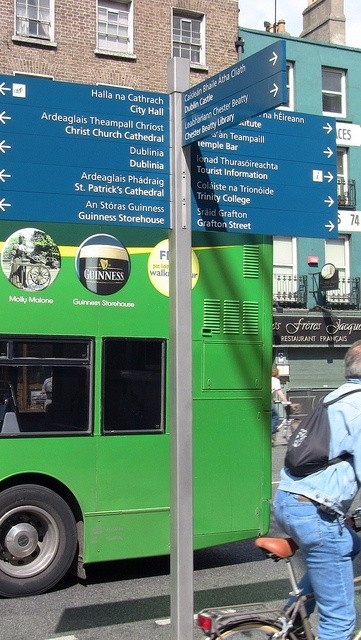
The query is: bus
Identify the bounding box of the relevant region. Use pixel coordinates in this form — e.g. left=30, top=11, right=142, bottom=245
left=1, top=219, right=270, bottom=597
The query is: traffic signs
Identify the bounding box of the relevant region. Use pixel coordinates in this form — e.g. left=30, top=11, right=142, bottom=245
left=1, top=40, right=338, bottom=241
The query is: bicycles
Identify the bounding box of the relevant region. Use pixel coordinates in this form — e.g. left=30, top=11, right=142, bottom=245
left=188, top=536, right=361, bottom=640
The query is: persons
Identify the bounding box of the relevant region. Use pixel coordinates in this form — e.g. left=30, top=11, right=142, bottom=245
left=272, top=339, right=361, bottom=640
left=271, top=368, right=292, bottom=446
